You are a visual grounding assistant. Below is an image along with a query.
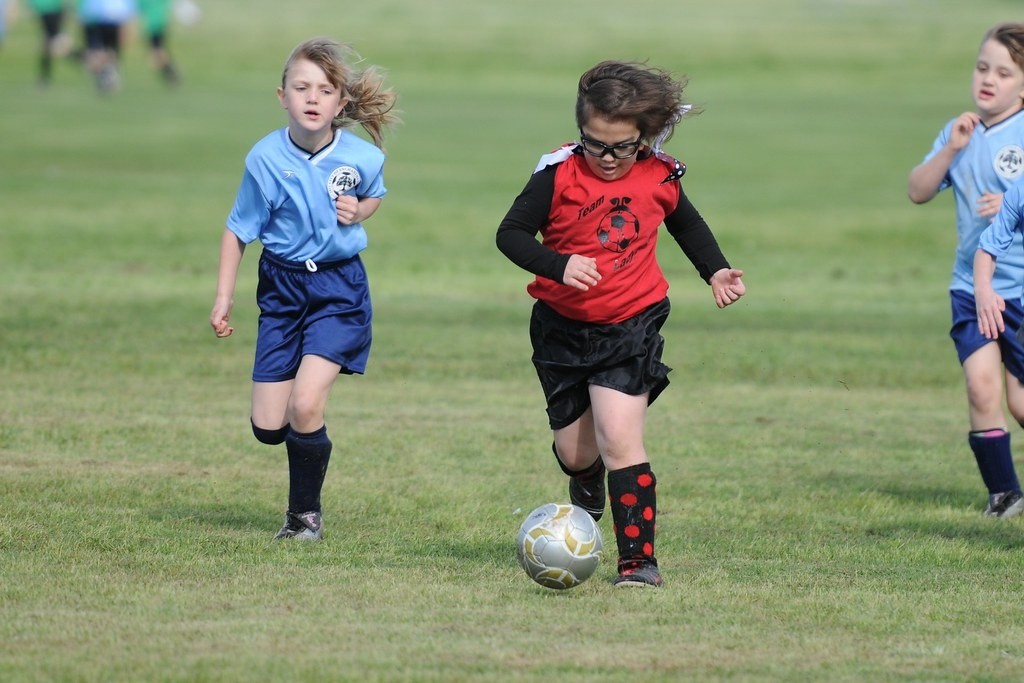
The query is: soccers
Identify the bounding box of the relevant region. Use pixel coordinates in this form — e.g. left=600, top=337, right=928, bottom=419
left=514, top=500, right=605, bottom=591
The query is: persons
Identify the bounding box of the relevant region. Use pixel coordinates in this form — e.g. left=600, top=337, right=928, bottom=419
left=495, top=60, right=747, bottom=588
left=907, top=21, right=1023, bottom=518
left=210, top=36, right=403, bottom=540
left=4, top=1, right=197, bottom=93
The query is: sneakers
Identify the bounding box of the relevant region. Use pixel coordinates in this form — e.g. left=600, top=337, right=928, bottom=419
left=613, top=554, right=663, bottom=588
left=569, top=477, right=607, bottom=522
left=982, top=492, right=1023, bottom=519
left=273, top=508, right=323, bottom=545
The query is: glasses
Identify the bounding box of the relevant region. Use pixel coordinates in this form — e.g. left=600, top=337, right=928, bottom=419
left=578, top=124, right=648, bottom=159
